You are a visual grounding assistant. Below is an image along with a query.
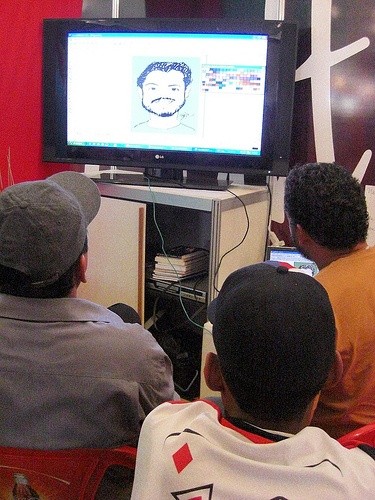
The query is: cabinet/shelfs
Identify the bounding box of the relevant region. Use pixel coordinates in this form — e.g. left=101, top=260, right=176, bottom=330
left=77, top=183, right=271, bottom=399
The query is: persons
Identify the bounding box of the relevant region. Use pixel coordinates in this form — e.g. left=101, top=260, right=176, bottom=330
left=0, top=172, right=181, bottom=500
left=285, top=164, right=374, bottom=438
left=132, top=260, right=375, bottom=500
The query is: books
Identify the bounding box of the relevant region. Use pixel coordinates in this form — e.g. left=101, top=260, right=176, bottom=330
left=153, top=246, right=209, bottom=282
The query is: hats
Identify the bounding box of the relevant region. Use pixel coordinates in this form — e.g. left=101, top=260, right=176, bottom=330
left=0, top=171, right=100, bottom=288
left=208, top=263, right=336, bottom=394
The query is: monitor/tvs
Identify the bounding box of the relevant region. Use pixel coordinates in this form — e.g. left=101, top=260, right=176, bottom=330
left=41, top=16, right=298, bottom=191
left=266, top=246, right=319, bottom=277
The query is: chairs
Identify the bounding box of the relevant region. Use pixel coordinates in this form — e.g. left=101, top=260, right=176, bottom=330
left=0, top=446, right=136, bottom=500
